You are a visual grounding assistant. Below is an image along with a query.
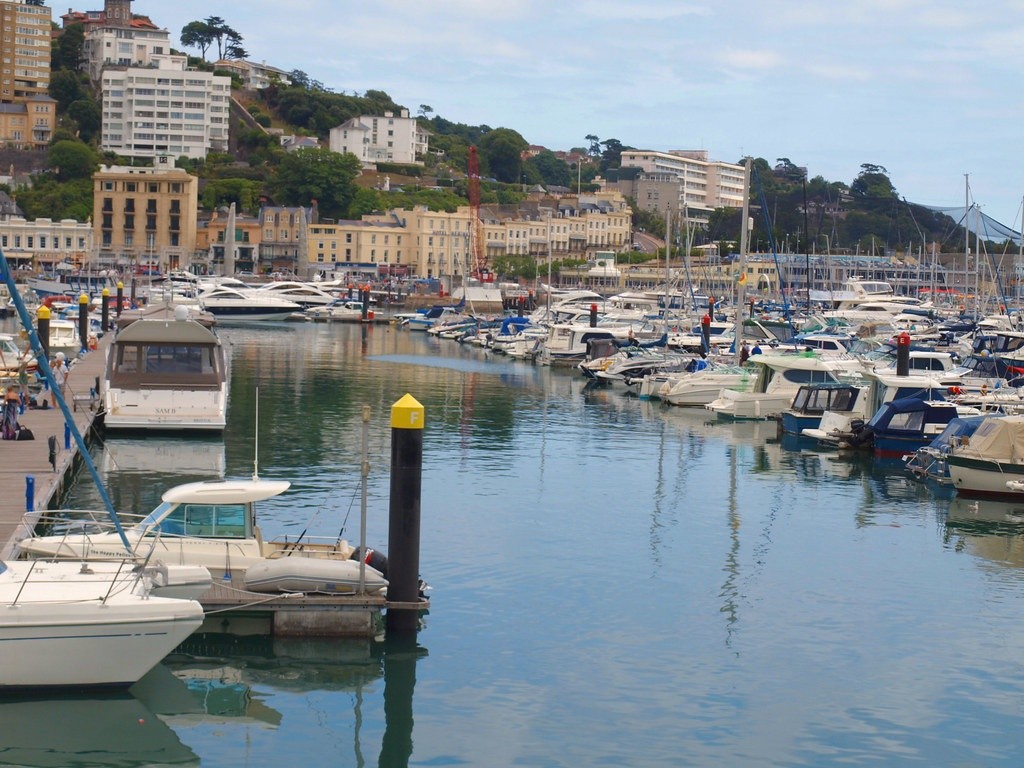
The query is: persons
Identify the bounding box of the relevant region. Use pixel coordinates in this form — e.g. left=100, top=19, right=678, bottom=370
left=741, top=340, right=762, bottom=363
left=19, top=364, right=29, bottom=405
left=711, top=343, right=721, bottom=356
left=3, top=386, right=21, bottom=430
left=50, top=358, right=69, bottom=408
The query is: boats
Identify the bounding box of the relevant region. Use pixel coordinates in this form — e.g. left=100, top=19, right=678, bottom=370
left=100, top=298, right=232, bottom=431
left=0, top=175, right=1024, bottom=503
left=947, top=494, right=1023, bottom=565
left=101, top=431, right=228, bottom=479
left=0, top=555, right=213, bottom=690
left=16, top=475, right=390, bottom=641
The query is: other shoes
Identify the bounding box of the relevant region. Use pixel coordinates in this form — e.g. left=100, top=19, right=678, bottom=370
left=48, top=405, right=57, bottom=409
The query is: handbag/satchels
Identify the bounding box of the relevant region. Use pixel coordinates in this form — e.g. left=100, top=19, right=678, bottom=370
left=2, top=423, right=16, bottom=440
left=0, top=417, right=21, bottom=432
left=17, top=425, right=35, bottom=441
